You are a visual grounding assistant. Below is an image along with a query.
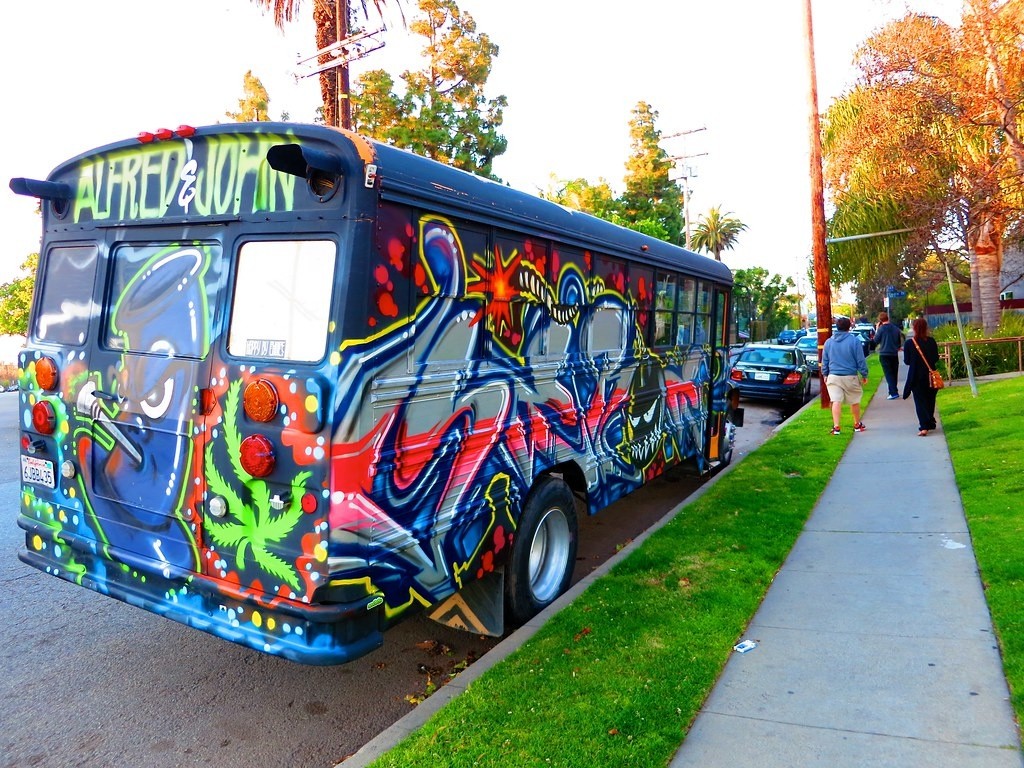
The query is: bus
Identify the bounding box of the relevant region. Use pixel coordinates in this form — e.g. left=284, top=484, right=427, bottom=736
left=9, top=122, right=746, bottom=667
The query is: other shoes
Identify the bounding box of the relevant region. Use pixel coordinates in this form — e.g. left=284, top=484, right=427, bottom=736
left=885, top=394, right=899, bottom=400
left=916, top=430, right=929, bottom=436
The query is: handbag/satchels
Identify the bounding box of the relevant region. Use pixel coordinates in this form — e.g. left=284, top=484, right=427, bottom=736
left=929, top=370, right=945, bottom=389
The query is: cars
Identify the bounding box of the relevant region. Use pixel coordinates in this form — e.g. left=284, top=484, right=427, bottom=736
left=729, top=318, right=881, bottom=408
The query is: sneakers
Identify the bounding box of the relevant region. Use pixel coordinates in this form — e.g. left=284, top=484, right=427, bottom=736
left=830, top=425, right=841, bottom=435
left=853, top=421, right=868, bottom=432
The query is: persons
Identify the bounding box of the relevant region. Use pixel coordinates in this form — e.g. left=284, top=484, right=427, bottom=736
left=873, top=312, right=902, bottom=400
left=903, top=318, right=939, bottom=436
left=821, top=318, right=868, bottom=435
